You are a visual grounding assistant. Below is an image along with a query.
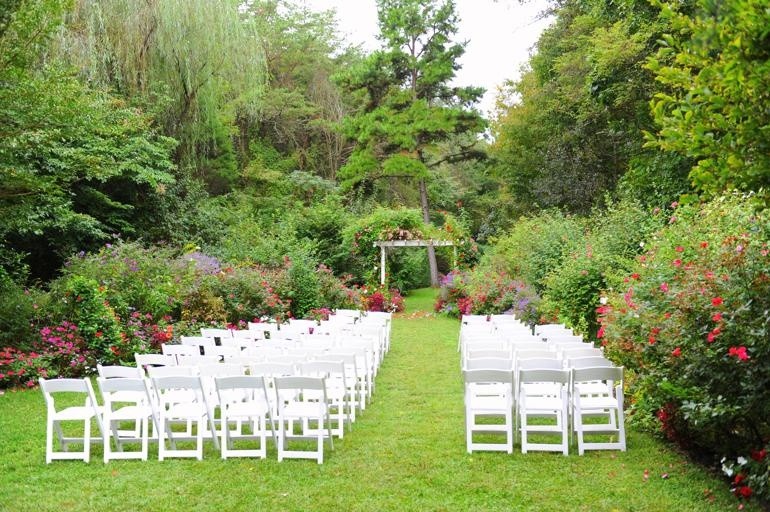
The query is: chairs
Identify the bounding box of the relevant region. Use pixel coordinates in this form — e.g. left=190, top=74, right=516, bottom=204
left=38, top=310, right=391, bottom=467
left=459, top=314, right=627, bottom=456
